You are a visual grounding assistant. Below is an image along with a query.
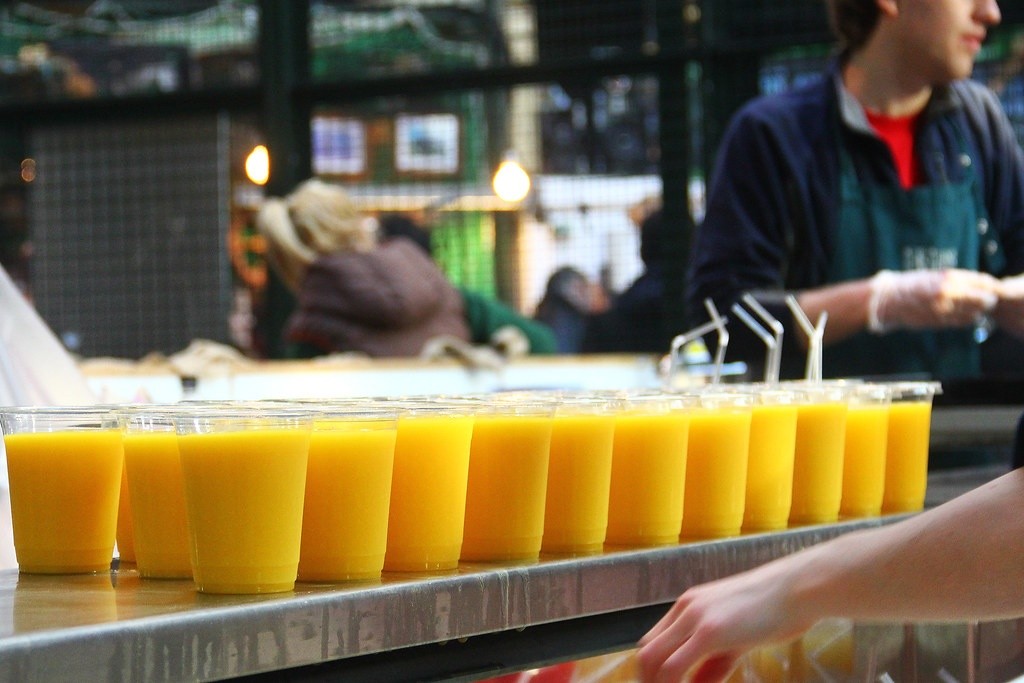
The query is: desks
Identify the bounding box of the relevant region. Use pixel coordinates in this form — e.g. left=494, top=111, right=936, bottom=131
left=0, top=464, right=1010, bottom=683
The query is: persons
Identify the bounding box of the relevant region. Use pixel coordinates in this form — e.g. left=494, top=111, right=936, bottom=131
left=257, top=180, right=555, bottom=361
left=638, top=465, right=1024, bottom=683
left=537, top=211, right=665, bottom=354
left=688, top=0, right=1024, bottom=400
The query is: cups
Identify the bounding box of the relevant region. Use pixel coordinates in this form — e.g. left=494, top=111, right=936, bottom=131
left=0, top=373, right=944, bottom=598
